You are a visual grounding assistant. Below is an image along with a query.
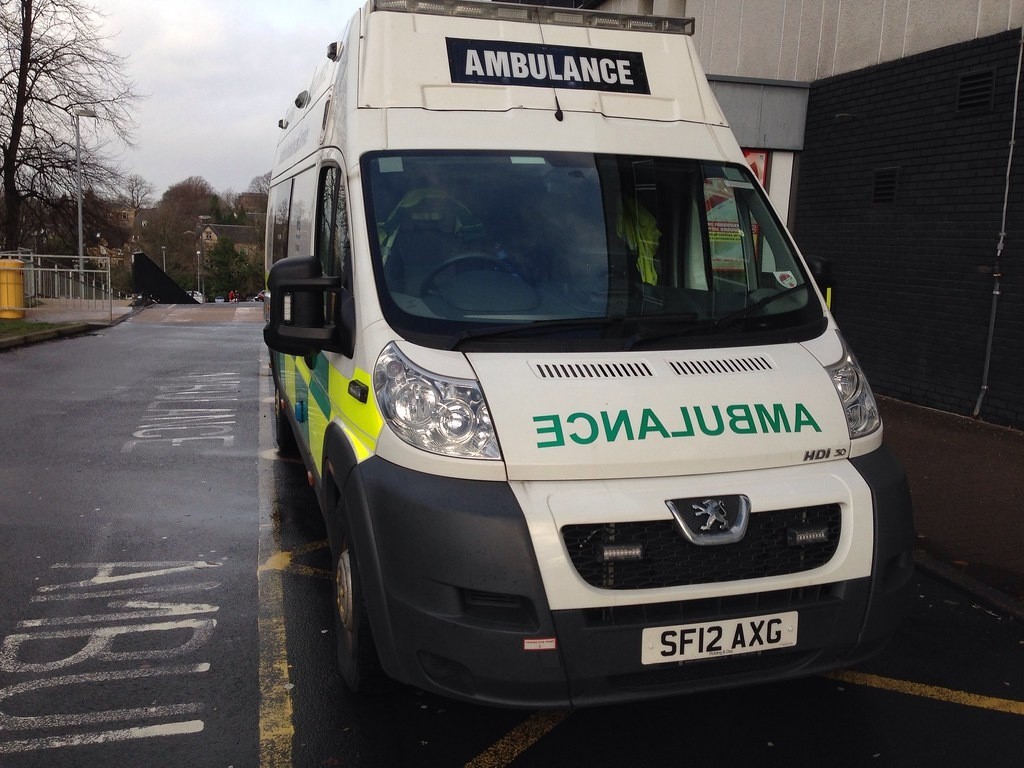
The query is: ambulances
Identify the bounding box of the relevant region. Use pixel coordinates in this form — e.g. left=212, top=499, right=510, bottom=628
left=262, top=0, right=919, bottom=712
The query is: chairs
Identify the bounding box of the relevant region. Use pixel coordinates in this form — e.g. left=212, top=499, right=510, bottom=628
left=385, top=187, right=475, bottom=299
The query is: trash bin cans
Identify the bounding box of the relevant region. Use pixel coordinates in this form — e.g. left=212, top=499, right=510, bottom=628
left=0, top=259, right=26, bottom=319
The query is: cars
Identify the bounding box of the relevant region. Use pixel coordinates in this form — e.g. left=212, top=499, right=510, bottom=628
left=186, top=290, right=203, bottom=303
left=214, top=295, right=226, bottom=302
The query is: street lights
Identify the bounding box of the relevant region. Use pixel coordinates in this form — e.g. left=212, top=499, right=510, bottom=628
left=161, top=246, right=167, bottom=273
left=73, top=109, right=96, bottom=299
left=196, top=251, right=201, bottom=303
